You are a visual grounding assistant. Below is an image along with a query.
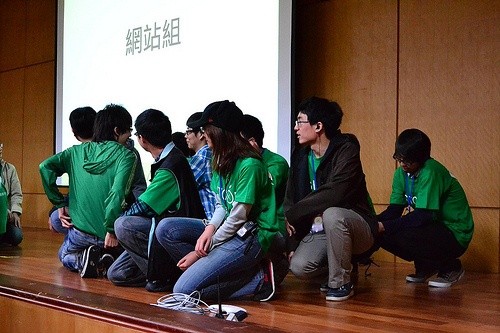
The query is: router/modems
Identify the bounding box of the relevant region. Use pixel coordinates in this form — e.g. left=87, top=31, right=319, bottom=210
left=207, top=273, right=248, bottom=323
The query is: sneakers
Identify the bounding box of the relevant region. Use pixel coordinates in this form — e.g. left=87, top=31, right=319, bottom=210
left=252, top=258, right=276, bottom=301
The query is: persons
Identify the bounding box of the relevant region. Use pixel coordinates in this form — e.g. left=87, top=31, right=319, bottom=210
left=285, top=98, right=379, bottom=301
left=39, top=100, right=289, bottom=302
left=376, top=129, right=474, bottom=288
left=0, top=143, right=24, bottom=247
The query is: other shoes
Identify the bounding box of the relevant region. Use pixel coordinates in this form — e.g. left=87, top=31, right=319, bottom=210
left=78, top=245, right=100, bottom=278
left=145, top=273, right=181, bottom=292
left=325, top=281, right=355, bottom=301
left=406, top=264, right=437, bottom=283
left=428, top=258, right=466, bottom=288
left=97, top=248, right=115, bottom=277
left=320, top=278, right=330, bottom=291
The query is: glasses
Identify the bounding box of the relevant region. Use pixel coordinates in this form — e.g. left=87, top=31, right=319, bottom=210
left=129, top=128, right=133, bottom=132
left=393, top=152, right=412, bottom=164
left=295, top=120, right=310, bottom=128
left=186, top=130, right=194, bottom=135
left=134, top=132, right=139, bottom=136
left=200, top=126, right=207, bottom=133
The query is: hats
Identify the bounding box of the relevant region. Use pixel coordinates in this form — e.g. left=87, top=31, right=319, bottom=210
left=203, top=100, right=244, bottom=134
left=242, top=114, right=265, bottom=142
left=186, top=112, right=204, bottom=129
